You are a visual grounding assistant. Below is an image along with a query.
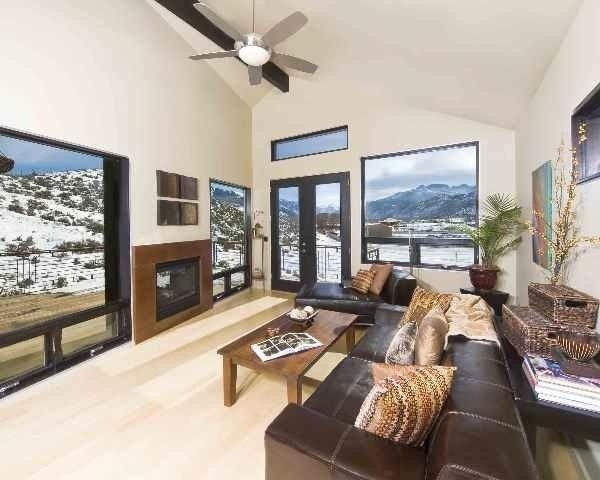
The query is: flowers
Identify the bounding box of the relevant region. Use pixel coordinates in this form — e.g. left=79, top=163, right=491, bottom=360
left=526, top=119, right=600, bottom=284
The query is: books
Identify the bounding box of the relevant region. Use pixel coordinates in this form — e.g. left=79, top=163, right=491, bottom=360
left=251, top=332, right=325, bottom=363
left=521, top=350, right=600, bottom=415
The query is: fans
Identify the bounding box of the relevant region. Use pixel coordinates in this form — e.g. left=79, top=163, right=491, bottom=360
left=184, top=1, right=320, bottom=87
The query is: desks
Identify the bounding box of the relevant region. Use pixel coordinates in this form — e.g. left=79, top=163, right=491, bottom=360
left=493, top=315, right=600, bottom=463
left=460, top=285, right=510, bottom=316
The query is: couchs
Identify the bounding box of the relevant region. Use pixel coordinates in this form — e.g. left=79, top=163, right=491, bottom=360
left=262, top=303, right=538, bottom=479
left=295, top=270, right=418, bottom=327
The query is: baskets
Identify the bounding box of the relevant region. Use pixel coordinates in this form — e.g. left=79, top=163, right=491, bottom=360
left=556, top=331, right=600, bottom=361
left=501, top=303, right=590, bottom=353
left=529, top=283, right=600, bottom=328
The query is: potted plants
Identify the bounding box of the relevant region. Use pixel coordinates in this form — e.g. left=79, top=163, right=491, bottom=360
left=451, top=189, right=528, bottom=290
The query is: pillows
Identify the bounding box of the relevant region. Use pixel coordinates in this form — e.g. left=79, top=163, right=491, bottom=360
left=368, top=263, right=394, bottom=296
left=349, top=367, right=452, bottom=449
left=352, top=269, right=376, bottom=294
left=414, top=306, right=451, bottom=366
left=398, top=285, right=452, bottom=328
left=386, top=319, right=417, bottom=366
left=366, top=362, right=458, bottom=386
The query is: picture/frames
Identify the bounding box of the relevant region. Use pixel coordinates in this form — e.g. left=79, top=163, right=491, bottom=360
left=568, top=82, right=600, bottom=186
